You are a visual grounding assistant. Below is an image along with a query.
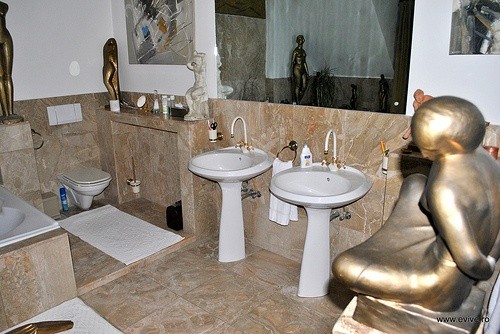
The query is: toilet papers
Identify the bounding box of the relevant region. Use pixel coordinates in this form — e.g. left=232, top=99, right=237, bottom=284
left=235, top=151, right=382, bottom=255
